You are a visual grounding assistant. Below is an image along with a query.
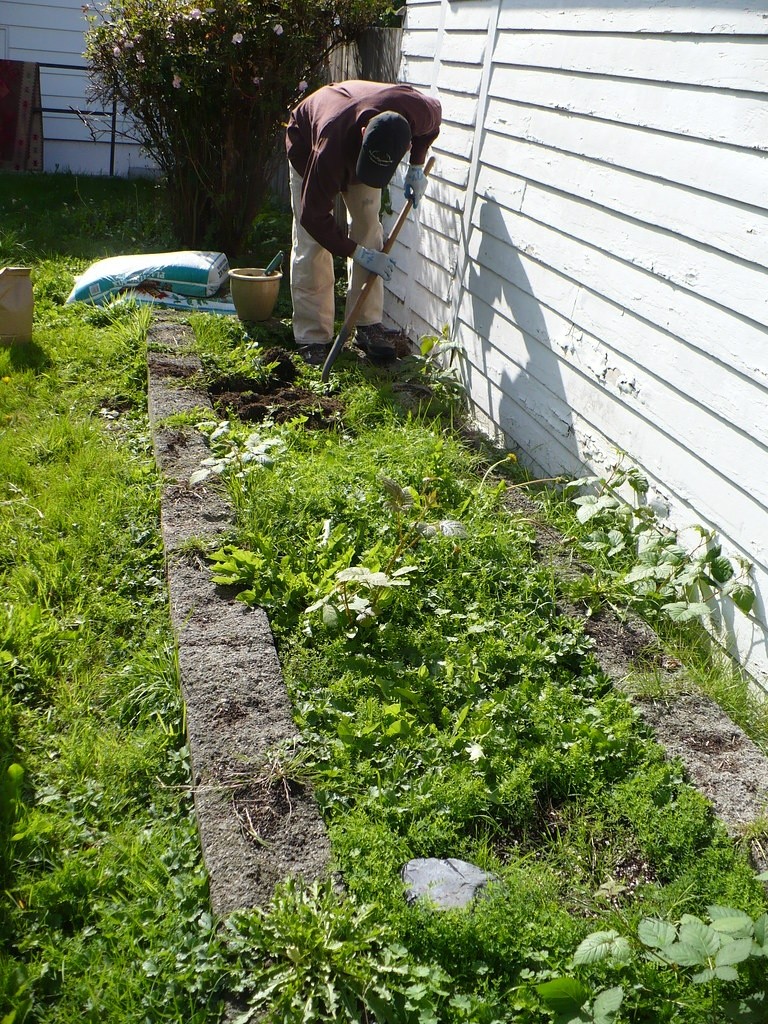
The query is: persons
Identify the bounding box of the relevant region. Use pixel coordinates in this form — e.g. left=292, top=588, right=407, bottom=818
left=285, top=80, right=441, bottom=365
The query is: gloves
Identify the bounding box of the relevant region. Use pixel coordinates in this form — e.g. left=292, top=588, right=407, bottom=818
left=353, top=244, right=395, bottom=281
left=404, top=164, right=427, bottom=209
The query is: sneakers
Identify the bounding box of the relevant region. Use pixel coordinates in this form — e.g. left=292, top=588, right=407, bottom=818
left=298, top=344, right=325, bottom=364
left=351, top=325, right=396, bottom=359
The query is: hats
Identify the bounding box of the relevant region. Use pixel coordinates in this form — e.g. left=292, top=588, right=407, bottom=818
left=356, top=111, right=411, bottom=188
